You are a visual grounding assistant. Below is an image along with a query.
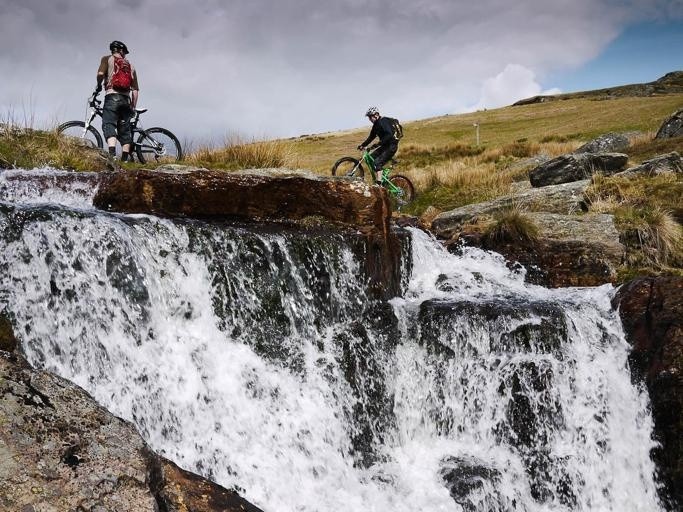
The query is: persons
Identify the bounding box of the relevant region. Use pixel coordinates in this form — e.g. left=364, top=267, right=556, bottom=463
left=356, top=107, right=399, bottom=187
left=95, top=41, right=139, bottom=163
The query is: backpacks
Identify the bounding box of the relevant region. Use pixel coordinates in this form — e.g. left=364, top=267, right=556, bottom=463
left=385, top=117, right=404, bottom=143
left=105, top=54, right=132, bottom=94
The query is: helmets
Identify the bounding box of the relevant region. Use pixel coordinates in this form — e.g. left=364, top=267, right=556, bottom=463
left=365, top=107, right=379, bottom=116
left=109, top=40, right=129, bottom=55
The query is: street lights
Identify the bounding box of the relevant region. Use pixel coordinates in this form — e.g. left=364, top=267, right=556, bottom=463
left=473, top=122, right=479, bottom=144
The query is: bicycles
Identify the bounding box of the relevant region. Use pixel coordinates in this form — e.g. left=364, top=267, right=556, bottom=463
left=58, top=89, right=181, bottom=164
left=332, top=146, right=414, bottom=206
